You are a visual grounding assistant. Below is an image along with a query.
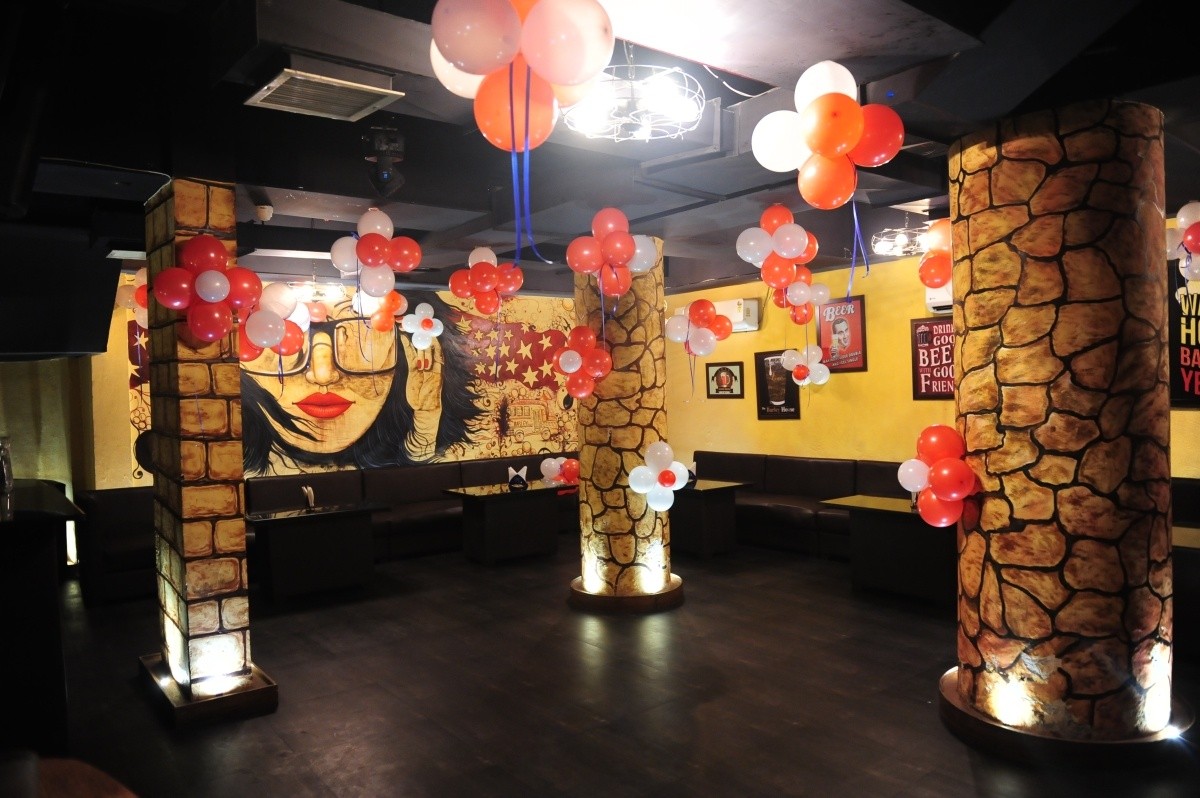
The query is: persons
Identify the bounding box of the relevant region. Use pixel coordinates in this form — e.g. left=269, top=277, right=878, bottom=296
left=828, top=318, right=850, bottom=359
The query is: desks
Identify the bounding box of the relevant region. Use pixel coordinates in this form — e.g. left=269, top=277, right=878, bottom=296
left=670, top=479, right=757, bottom=563
left=438, top=478, right=579, bottom=567
left=244, top=500, right=392, bottom=615
left=1171, top=524, right=1200, bottom=553
left=817, top=494, right=957, bottom=613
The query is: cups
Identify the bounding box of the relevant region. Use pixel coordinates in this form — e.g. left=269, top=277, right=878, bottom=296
left=831, top=334, right=838, bottom=358
left=764, top=356, right=786, bottom=405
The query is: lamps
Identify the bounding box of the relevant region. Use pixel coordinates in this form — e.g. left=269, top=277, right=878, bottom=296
left=284, top=271, right=347, bottom=304
left=559, top=36, right=705, bottom=144
left=372, top=154, right=405, bottom=209
left=870, top=210, right=926, bottom=257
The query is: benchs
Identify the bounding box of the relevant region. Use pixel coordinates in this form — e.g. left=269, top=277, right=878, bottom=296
left=74, top=450, right=579, bottom=610
left=693, top=450, right=1199, bottom=666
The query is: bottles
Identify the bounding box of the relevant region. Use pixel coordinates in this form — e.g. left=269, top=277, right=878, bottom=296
left=0, top=437, right=14, bottom=493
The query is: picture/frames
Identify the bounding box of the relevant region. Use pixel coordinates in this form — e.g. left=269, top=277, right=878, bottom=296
left=706, top=361, right=745, bottom=399
left=1166, top=258, right=1200, bottom=409
left=754, top=349, right=801, bottom=420
left=910, top=316, right=955, bottom=401
left=815, top=294, right=869, bottom=373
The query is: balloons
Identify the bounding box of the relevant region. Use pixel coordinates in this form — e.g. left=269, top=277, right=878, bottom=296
left=114, top=0, right=974, bottom=528
left=1165, top=201, right=1200, bottom=280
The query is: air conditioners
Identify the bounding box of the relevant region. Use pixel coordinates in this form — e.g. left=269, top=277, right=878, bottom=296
left=675, top=298, right=761, bottom=333
left=924, top=277, right=953, bottom=314
